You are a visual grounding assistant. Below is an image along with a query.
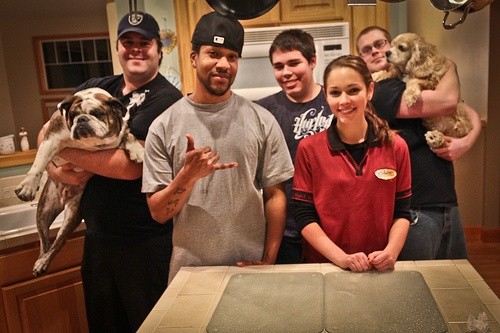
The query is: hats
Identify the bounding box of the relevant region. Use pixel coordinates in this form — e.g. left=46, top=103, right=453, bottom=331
left=191, top=12, right=244, bottom=54
left=117, top=11, right=159, bottom=39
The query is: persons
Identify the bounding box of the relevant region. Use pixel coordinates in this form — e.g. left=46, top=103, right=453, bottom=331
left=290, top=54, right=413, bottom=272
left=141, top=13, right=295, bottom=285
left=252, top=29, right=336, bottom=263
left=36, top=11, right=181, bottom=333
left=355, top=25, right=482, bottom=261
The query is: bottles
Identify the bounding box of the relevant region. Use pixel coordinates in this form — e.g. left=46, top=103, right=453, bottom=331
left=19, top=125, right=29, bottom=152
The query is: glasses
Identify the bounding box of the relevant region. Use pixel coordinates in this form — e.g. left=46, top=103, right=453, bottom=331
left=359, top=39, right=388, bottom=57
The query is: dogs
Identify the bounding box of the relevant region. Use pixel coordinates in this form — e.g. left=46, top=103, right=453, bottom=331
left=371, top=33, right=473, bottom=152
left=15, top=86, right=145, bottom=277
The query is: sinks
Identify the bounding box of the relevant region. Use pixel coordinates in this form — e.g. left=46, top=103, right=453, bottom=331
left=0, top=201, right=65, bottom=236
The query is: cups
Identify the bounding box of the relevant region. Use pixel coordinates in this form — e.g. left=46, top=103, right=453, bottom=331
left=0, top=134, right=16, bottom=155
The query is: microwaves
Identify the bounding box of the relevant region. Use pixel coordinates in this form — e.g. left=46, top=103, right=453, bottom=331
left=228, top=22, right=351, bottom=101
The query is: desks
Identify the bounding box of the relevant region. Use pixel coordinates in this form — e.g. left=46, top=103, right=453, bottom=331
left=136, top=259, right=499, bottom=333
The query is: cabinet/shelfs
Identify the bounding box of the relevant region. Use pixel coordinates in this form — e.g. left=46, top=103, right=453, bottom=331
left=0, top=228, right=88, bottom=333
left=175, top=0, right=390, bottom=98
